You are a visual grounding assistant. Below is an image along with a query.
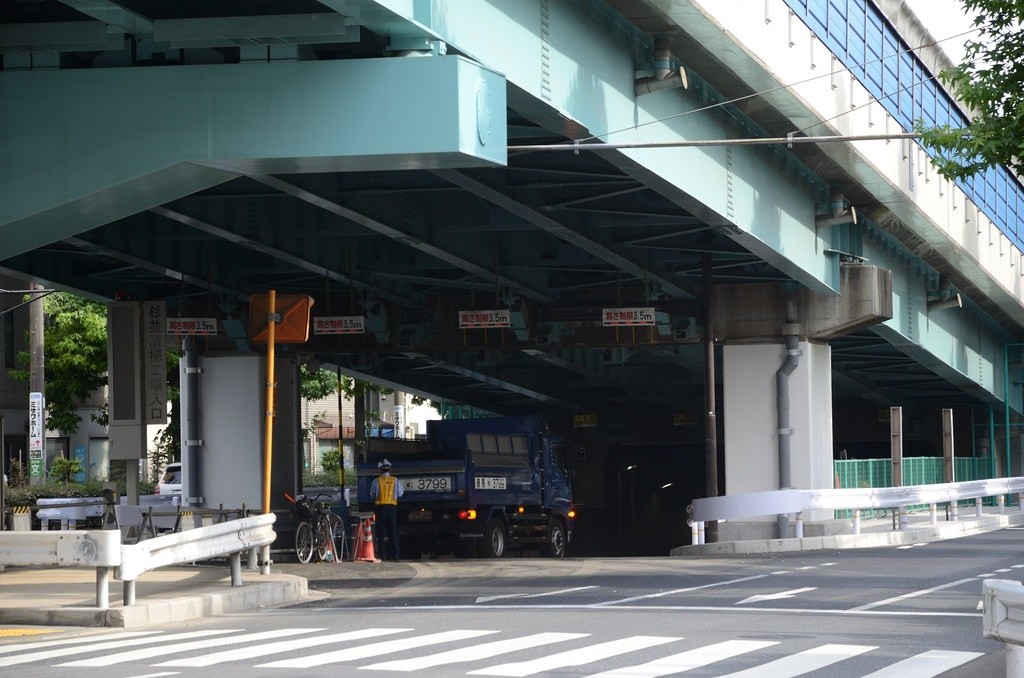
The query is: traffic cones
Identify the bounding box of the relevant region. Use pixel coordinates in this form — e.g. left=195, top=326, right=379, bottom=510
left=354, top=521, right=363, bottom=560
left=359, top=518, right=382, bottom=562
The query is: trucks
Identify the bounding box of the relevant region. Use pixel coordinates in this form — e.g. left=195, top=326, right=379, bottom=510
left=356, top=415, right=585, bottom=559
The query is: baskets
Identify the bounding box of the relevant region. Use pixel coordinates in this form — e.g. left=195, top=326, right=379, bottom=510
left=289, top=501, right=315, bottom=520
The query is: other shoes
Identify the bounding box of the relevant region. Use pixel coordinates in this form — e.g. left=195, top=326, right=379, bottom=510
left=378, top=556, right=386, bottom=560
left=393, top=556, right=399, bottom=560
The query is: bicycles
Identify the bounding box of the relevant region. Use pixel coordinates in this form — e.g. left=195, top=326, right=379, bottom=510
left=292, top=492, right=333, bottom=564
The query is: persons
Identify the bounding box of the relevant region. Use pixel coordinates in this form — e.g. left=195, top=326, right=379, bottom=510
left=369, top=459, right=404, bottom=560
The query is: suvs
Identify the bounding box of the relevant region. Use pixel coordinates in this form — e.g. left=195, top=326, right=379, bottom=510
left=154, top=462, right=181, bottom=498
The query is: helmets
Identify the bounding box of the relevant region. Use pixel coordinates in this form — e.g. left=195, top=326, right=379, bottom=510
left=378, top=458, right=392, bottom=468
